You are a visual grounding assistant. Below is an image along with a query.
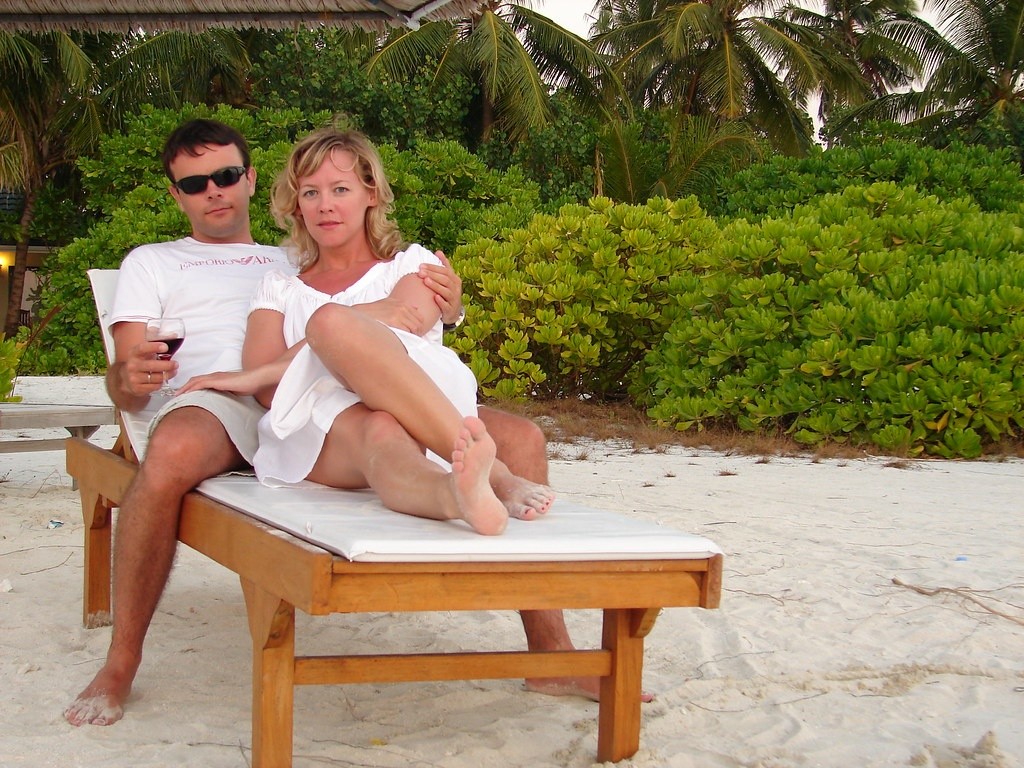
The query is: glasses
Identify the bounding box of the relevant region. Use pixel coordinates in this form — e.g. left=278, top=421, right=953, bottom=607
left=174, top=166, right=246, bottom=195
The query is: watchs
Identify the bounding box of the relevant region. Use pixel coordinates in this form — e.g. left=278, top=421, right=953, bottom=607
left=443, top=305, right=466, bottom=329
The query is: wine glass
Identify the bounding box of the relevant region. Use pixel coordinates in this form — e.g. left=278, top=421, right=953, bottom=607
left=145, top=318, right=185, bottom=397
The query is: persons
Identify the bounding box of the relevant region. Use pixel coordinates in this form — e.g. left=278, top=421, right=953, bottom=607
left=174, top=112, right=555, bottom=535
left=62, top=118, right=655, bottom=732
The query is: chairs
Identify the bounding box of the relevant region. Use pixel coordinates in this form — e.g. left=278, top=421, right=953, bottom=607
left=65, top=268, right=724, bottom=768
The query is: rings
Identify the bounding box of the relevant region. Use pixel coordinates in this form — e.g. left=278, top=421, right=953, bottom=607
left=148, top=372, right=151, bottom=383
